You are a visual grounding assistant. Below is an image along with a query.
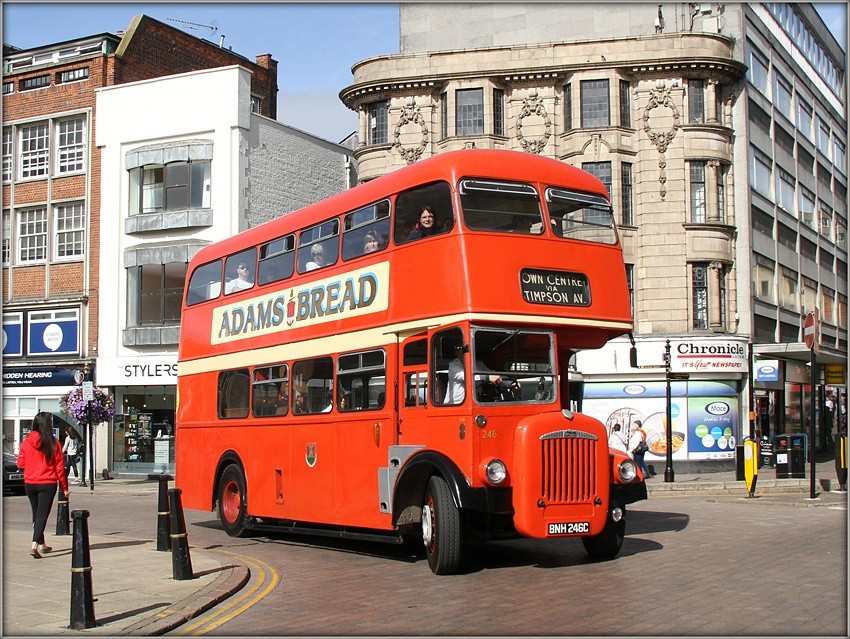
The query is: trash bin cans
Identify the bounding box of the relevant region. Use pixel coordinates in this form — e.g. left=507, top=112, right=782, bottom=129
left=776, top=432, right=808, bottom=479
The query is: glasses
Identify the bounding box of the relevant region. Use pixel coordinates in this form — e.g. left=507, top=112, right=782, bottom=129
left=455, top=346, right=462, bottom=351
left=312, top=252, right=322, bottom=257
left=237, top=265, right=249, bottom=270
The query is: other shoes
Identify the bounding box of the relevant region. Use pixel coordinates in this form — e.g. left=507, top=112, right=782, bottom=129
left=74, top=478, right=79, bottom=481
left=38, top=547, right=52, bottom=553
left=30, top=548, right=42, bottom=558
left=644, top=473, right=652, bottom=479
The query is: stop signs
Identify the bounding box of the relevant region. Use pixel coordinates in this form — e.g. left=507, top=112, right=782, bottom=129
left=804, top=313, right=815, bottom=349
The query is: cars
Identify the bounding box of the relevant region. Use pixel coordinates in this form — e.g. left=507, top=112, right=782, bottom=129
left=3, top=445, right=27, bottom=496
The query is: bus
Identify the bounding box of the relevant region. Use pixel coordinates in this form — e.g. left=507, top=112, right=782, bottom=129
left=171, top=148, right=649, bottom=577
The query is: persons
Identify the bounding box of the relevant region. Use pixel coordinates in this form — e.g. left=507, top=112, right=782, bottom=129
left=321, top=389, right=347, bottom=413
left=399, top=203, right=453, bottom=243
left=62, top=426, right=79, bottom=482
left=306, top=243, right=335, bottom=272
left=608, top=420, right=651, bottom=479
left=363, top=229, right=385, bottom=254
left=228, top=261, right=255, bottom=294
left=162, top=418, right=172, bottom=435
left=824, top=394, right=836, bottom=447
left=16, top=412, right=70, bottom=558
left=443, top=336, right=522, bottom=404
left=511, top=211, right=533, bottom=234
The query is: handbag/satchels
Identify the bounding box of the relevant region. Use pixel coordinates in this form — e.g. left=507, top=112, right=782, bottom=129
left=632, top=442, right=648, bottom=455
